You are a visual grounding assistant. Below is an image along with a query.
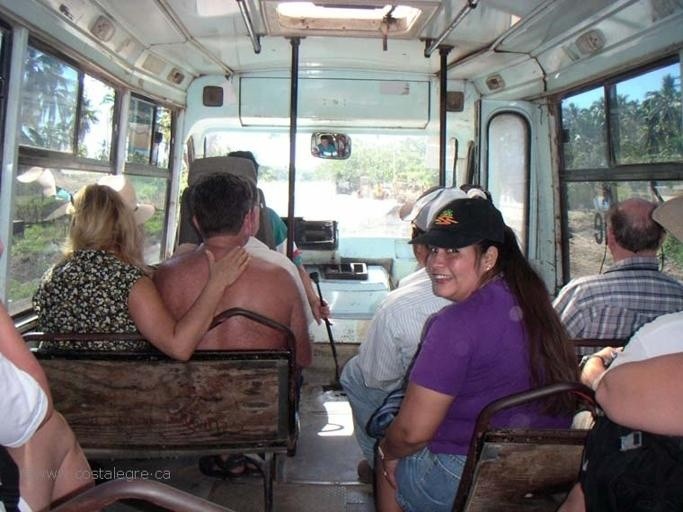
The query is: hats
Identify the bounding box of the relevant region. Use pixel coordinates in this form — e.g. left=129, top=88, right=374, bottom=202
left=11, top=167, right=74, bottom=223
left=97, top=175, right=155, bottom=225
left=404, top=187, right=504, bottom=249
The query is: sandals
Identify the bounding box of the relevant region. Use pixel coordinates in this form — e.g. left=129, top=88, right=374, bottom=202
left=199, top=455, right=249, bottom=479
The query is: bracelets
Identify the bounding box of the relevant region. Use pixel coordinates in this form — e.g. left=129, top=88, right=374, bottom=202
left=373, top=436, right=397, bottom=461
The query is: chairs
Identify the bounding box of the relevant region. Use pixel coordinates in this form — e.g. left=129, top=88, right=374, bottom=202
left=174, top=156, right=276, bottom=248
left=453, top=382, right=610, bottom=511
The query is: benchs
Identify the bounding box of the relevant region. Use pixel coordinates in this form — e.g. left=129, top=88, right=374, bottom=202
left=565, top=336, right=634, bottom=372
left=22, top=307, right=303, bottom=511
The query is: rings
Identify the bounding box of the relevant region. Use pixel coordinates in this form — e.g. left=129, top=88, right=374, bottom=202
left=381, top=468, right=388, bottom=476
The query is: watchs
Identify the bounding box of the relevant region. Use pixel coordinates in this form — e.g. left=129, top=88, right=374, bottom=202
left=576, top=352, right=607, bottom=373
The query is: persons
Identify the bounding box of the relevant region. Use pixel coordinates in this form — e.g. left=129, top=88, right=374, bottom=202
left=554, top=350, right=682, bottom=512
left=372, top=194, right=580, bottom=510
left=0, top=299, right=97, bottom=511
left=577, top=192, right=682, bottom=392
left=339, top=186, right=470, bottom=484
left=30, top=182, right=252, bottom=463
left=225, top=151, right=333, bottom=326
left=195, top=172, right=312, bottom=457
left=151, top=169, right=313, bottom=480
left=313, top=133, right=348, bottom=159
left=460, top=182, right=494, bottom=206
left=548, top=195, right=683, bottom=363
